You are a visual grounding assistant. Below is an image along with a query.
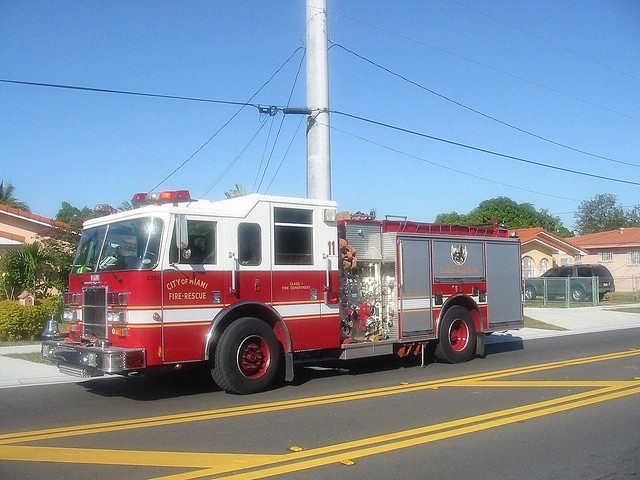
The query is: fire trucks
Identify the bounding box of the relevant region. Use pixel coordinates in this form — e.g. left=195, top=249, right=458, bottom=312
left=43, top=190, right=525, bottom=394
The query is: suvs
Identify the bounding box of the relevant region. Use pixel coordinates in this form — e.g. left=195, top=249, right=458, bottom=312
left=524, top=264, right=616, bottom=302
